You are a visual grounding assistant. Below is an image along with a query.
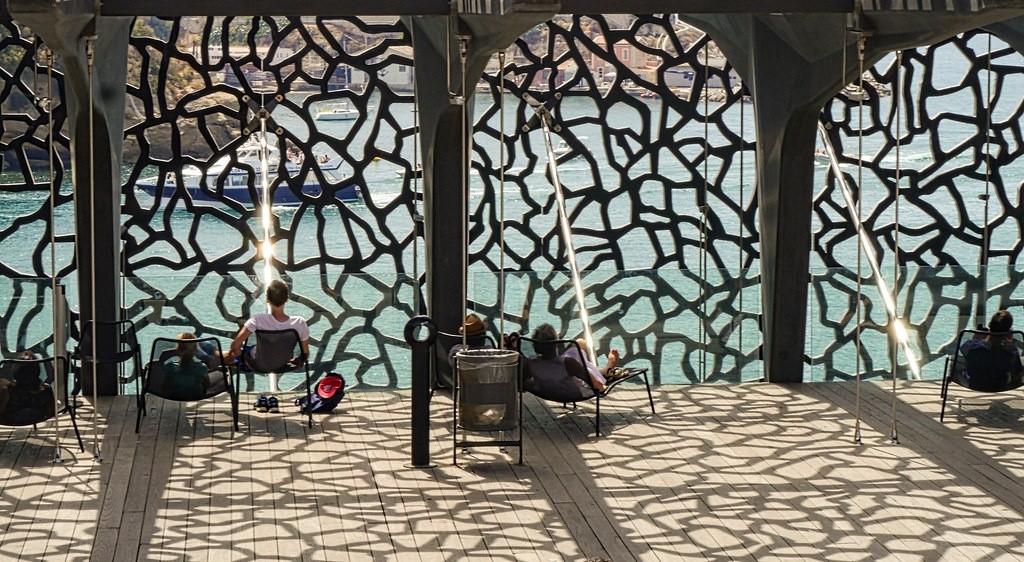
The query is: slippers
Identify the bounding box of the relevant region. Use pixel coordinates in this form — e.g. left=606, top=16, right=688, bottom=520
left=604, top=367, right=618, bottom=379
left=606, top=368, right=629, bottom=382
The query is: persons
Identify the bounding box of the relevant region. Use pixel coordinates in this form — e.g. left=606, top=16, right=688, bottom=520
left=231, top=280, right=309, bottom=370
left=168, top=174, right=172, bottom=183
left=529, top=323, right=620, bottom=402
left=448, top=314, right=520, bottom=402
left=164, top=333, right=235, bottom=400
left=816, top=149, right=825, bottom=154
left=287, top=147, right=328, bottom=163
left=960, top=310, right=1014, bottom=384
left=0, top=350, right=49, bottom=424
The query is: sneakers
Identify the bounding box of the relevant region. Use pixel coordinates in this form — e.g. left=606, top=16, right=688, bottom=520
left=268, top=397, right=279, bottom=413
left=254, top=394, right=268, bottom=412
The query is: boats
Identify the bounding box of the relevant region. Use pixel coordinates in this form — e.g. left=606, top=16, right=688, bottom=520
left=134, top=131, right=364, bottom=209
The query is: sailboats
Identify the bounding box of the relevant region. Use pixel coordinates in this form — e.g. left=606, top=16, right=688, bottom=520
left=313, top=39, right=373, bottom=121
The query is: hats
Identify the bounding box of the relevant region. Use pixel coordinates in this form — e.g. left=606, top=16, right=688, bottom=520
left=458, top=314, right=489, bottom=336
left=318, top=376, right=341, bottom=398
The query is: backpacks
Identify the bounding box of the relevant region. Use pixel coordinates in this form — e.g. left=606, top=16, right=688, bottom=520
left=295, top=373, right=345, bottom=415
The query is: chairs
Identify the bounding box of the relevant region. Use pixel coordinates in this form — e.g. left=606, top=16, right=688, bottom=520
left=65, top=318, right=146, bottom=416
left=224, top=329, right=313, bottom=428
left=939, top=330, right=1024, bottom=424
left=0, top=356, right=85, bottom=454
left=510, top=336, right=655, bottom=439
left=428, top=331, right=517, bottom=402
left=135, top=337, right=239, bottom=434
